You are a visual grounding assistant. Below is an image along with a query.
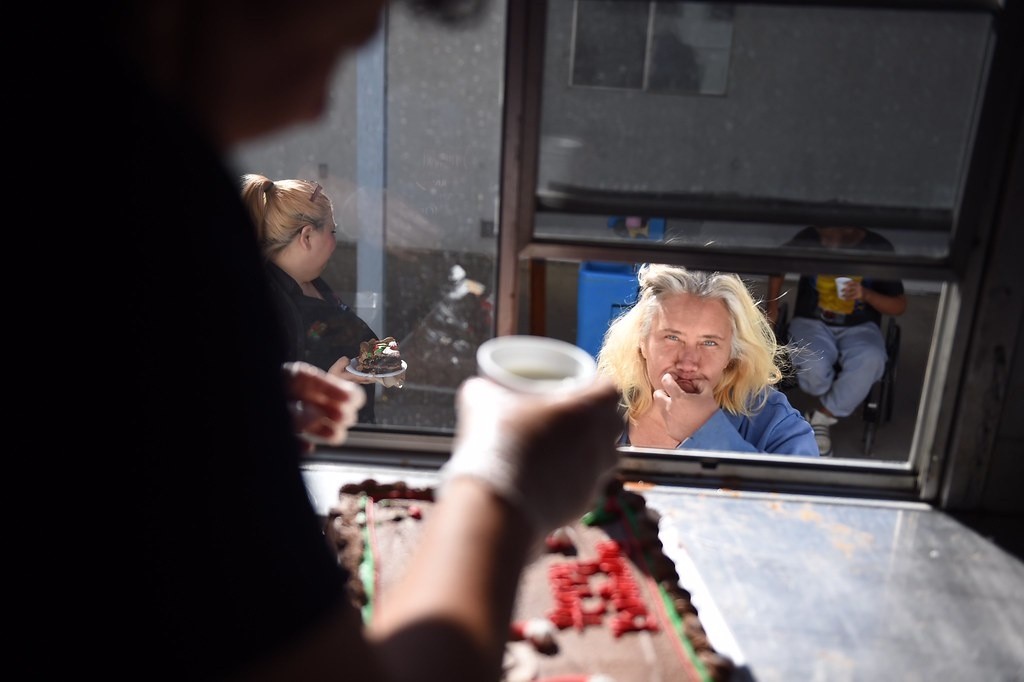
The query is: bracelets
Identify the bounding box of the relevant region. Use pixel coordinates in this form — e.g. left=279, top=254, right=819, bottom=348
left=440, top=451, right=547, bottom=567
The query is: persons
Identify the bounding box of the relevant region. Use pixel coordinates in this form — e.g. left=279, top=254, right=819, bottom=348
left=0, top=0, right=626, bottom=682
left=765, top=224, right=907, bottom=457
left=239, top=174, right=380, bottom=424
left=597, top=261, right=820, bottom=458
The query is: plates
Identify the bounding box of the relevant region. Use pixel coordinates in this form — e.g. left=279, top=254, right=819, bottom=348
left=348, top=359, right=408, bottom=378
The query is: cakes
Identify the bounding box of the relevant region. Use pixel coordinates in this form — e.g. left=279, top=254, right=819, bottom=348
left=329, top=479, right=744, bottom=682
left=354, top=337, right=403, bottom=374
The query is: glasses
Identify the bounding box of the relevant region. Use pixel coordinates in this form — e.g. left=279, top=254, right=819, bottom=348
left=299, top=178, right=322, bottom=202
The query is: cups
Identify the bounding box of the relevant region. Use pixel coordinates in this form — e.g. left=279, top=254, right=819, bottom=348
left=475, top=334, right=597, bottom=391
left=835, top=276, right=851, bottom=299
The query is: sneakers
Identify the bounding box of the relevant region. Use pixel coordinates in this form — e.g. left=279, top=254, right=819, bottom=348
left=805, top=410, right=838, bottom=459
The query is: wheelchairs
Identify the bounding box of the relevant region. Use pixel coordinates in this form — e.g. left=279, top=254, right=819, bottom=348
left=770, top=300, right=902, bottom=461
